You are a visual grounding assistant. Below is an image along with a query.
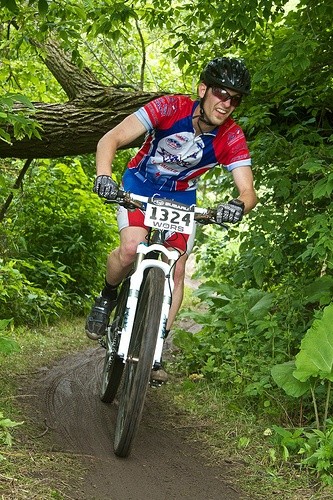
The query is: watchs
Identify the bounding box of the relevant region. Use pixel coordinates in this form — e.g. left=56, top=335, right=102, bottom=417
left=228, top=197, right=245, bottom=209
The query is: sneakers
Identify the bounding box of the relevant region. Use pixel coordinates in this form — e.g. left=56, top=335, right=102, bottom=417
left=85, top=288, right=120, bottom=340
left=150, top=357, right=168, bottom=381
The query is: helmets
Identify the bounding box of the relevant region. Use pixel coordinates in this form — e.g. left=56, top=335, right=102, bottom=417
left=200, top=57, right=252, bottom=95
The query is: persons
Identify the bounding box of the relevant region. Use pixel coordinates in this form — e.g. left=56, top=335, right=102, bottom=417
left=85, top=59, right=259, bottom=387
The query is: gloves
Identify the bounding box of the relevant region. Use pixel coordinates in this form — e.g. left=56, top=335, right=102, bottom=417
left=215, top=198, right=245, bottom=225
left=93, top=174, right=119, bottom=200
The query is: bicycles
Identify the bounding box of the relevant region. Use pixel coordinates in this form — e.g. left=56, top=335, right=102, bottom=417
left=92, top=186, right=240, bottom=459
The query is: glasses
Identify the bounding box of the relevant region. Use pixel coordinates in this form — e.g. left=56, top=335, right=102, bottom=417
left=211, top=84, right=242, bottom=107
